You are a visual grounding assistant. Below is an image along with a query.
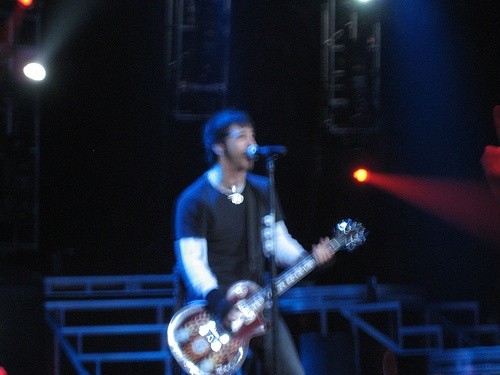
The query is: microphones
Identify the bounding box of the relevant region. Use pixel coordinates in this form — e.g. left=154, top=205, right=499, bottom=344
left=246, top=144, right=286, bottom=158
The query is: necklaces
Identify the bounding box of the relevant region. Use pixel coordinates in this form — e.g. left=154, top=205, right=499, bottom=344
left=210, top=170, right=249, bottom=204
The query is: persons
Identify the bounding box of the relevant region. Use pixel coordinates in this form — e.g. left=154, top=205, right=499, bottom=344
left=175, top=110, right=333, bottom=375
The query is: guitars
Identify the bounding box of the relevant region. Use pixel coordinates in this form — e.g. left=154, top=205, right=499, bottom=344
left=167, top=220, right=366, bottom=375
